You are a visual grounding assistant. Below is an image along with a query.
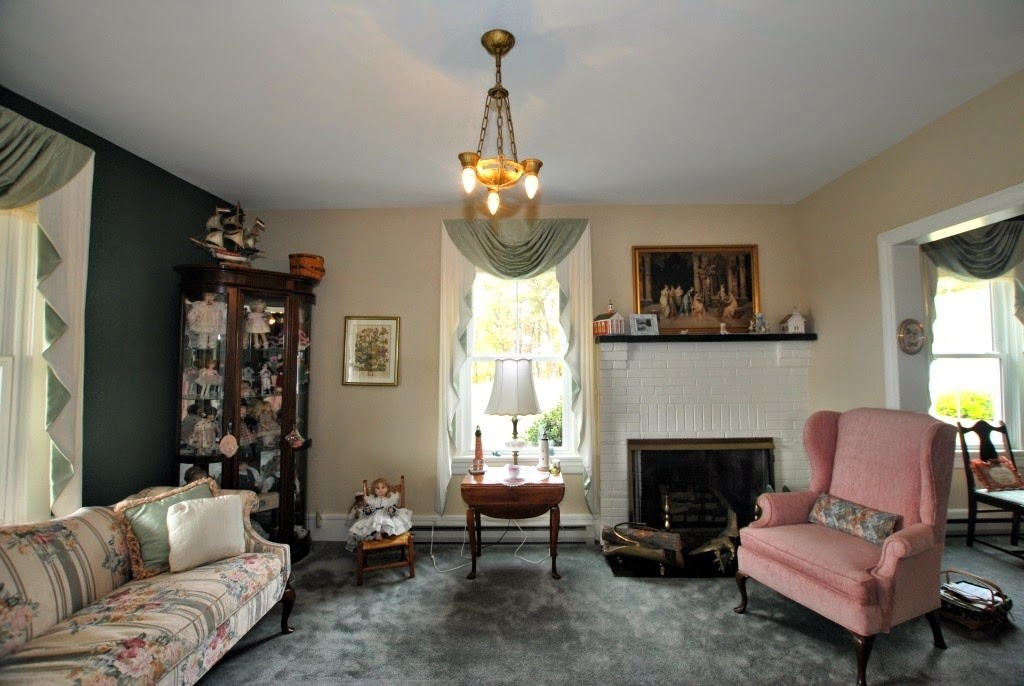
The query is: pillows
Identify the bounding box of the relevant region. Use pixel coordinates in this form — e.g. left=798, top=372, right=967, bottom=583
left=112, top=477, right=225, bottom=577
left=810, top=493, right=896, bottom=547
left=968, top=455, right=1024, bottom=491
left=168, top=494, right=244, bottom=572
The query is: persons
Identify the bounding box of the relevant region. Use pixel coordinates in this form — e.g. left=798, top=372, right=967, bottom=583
left=245, top=299, right=272, bottom=350
left=255, top=401, right=281, bottom=447
left=182, top=403, right=221, bottom=455
left=242, top=363, right=279, bottom=395
left=185, top=288, right=227, bottom=349
left=184, top=358, right=222, bottom=397
left=350, top=478, right=412, bottom=541
left=263, top=455, right=303, bottom=532
left=239, top=405, right=255, bottom=447
left=238, top=456, right=260, bottom=493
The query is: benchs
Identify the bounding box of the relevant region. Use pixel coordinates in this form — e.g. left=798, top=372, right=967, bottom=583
left=0, top=481, right=294, bottom=685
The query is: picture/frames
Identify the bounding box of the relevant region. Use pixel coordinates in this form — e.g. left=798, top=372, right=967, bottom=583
left=631, top=243, right=762, bottom=332
left=898, top=318, right=924, bottom=355
left=342, top=315, right=399, bottom=386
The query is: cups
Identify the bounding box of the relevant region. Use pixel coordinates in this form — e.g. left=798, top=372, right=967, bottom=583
left=509, top=465, right=518, bottom=477
left=473, top=458, right=483, bottom=470
left=552, top=458, right=560, bottom=468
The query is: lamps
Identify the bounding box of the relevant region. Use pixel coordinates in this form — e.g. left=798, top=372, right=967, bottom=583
left=486, top=358, right=542, bottom=482
left=458, top=28, right=543, bottom=214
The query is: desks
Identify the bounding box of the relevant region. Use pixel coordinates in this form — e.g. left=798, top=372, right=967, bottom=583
left=461, top=463, right=564, bottom=579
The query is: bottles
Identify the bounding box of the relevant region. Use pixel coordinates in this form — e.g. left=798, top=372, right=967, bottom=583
left=539, top=431, right=549, bottom=466
left=475, top=425, right=483, bottom=467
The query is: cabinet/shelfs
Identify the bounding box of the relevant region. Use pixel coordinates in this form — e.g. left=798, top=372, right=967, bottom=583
left=173, top=263, right=317, bottom=564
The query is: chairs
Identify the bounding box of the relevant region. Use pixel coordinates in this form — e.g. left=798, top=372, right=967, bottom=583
left=956, top=419, right=1024, bottom=560
left=355, top=474, right=414, bottom=588
left=737, top=405, right=959, bottom=686
